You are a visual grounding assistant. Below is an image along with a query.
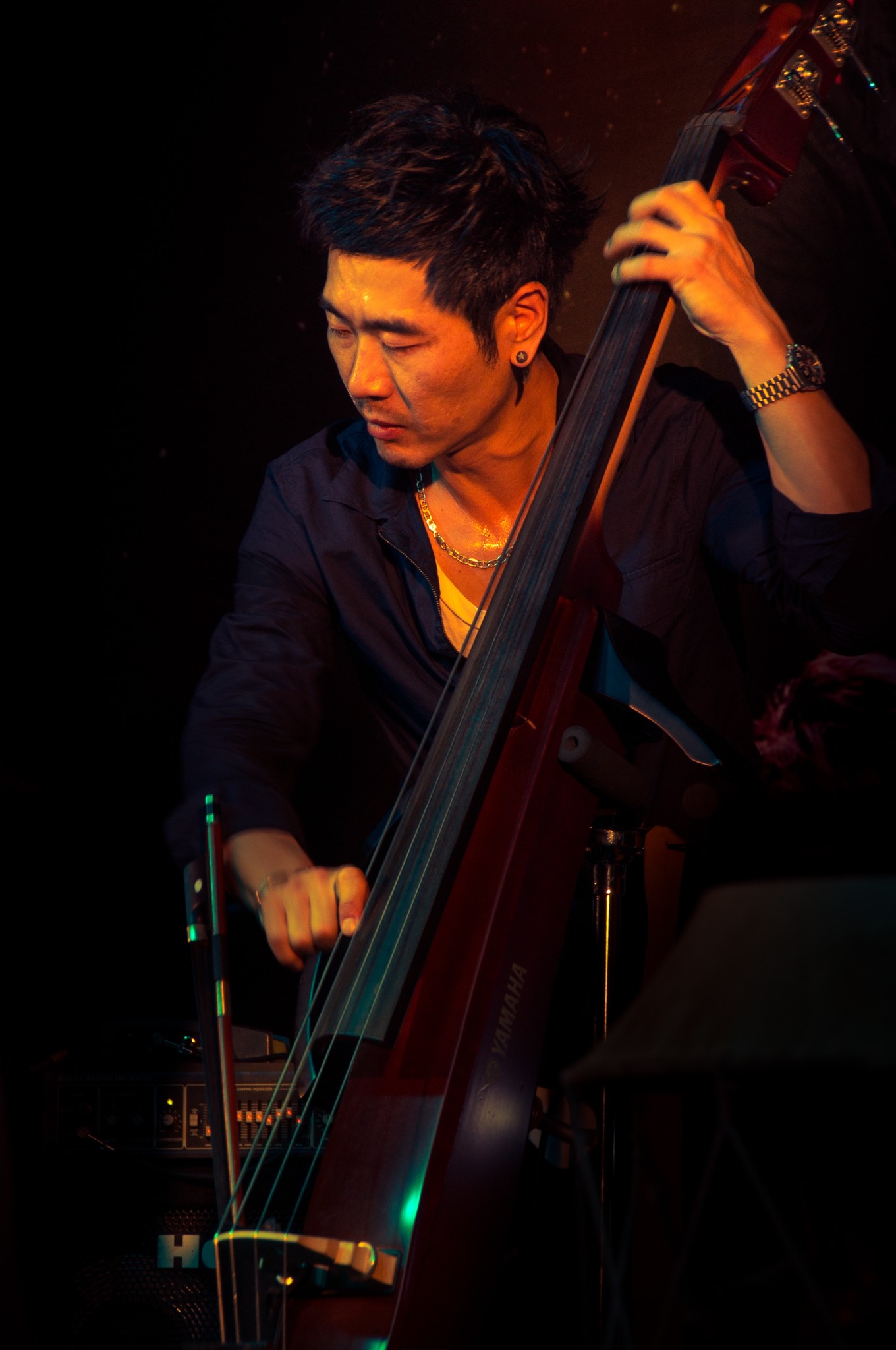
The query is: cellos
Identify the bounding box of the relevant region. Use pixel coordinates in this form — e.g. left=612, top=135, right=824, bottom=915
left=213, top=0, right=878, bottom=1350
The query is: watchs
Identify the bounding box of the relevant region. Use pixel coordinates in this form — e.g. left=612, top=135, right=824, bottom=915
left=737, top=341, right=826, bottom=413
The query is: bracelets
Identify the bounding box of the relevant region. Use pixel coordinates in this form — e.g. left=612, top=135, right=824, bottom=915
left=254, top=863, right=313, bottom=904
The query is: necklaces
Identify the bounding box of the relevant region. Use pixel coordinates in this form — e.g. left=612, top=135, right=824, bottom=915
left=414, top=465, right=513, bottom=568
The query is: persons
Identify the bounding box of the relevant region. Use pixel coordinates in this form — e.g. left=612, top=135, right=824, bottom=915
left=191, top=93, right=896, bottom=1350
